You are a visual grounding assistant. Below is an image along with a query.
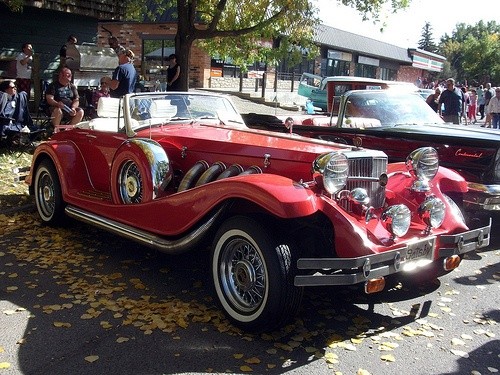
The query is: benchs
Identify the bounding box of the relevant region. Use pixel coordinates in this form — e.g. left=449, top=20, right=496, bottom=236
left=302, top=117, right=383, bottom=128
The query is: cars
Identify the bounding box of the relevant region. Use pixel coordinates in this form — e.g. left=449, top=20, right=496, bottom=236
left=24, top=89, right=494, bottom=336
left=266, top=71, right=500, bottom=239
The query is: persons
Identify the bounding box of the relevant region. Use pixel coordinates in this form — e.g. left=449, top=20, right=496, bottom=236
left=46, top=67, right=83, bottom=127
left=60, top=33, right=81, bottom=70
left=415, top=76, right=500, bottom=129
left=108, top=35, right=125, bottom=56
left=0, top=80, right=36, bottom=146
left=137, top=75, right=144, bottom=92
left=89, top=76, right=111, bottom=116
left=165, top=54, right=186, bottom=117
left=15, top=44, right=34, bottom=102
left=105, top=49, right=138, bottom=98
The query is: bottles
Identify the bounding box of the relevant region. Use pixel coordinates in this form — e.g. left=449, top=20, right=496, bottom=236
left=9, top=119, right=13, bottom=129
left=63, top=104, right=75, bottom=115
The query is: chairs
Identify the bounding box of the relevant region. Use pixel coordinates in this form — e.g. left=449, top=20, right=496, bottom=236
left=145, top=99, right=178, bottom=125
left=3, top=81, right=139, bottom=158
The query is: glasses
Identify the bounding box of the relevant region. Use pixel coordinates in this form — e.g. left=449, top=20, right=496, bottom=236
left=101, top=82, right=106, bottom=84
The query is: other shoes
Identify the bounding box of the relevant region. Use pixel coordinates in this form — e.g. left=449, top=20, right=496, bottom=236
left=479, top=125, right=485, bottom=127
